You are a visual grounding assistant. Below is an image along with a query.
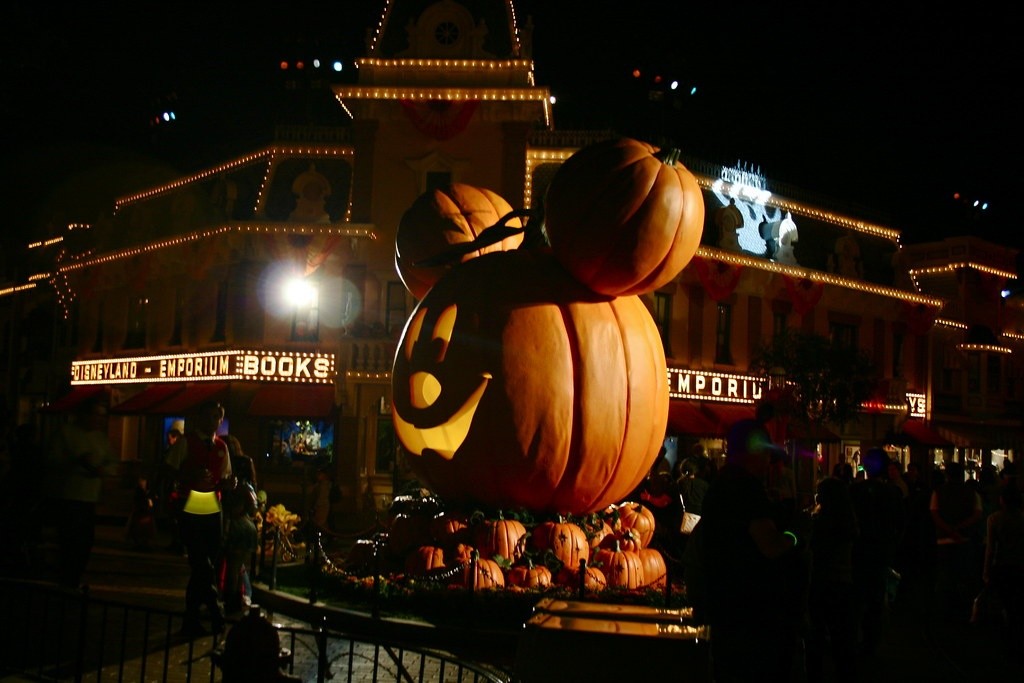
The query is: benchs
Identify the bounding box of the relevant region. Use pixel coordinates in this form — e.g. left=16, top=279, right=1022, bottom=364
left=524, top=599, right=710, bottom=675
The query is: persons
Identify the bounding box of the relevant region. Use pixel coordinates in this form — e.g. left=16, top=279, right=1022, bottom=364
left=0, top=392, right=1024, bottom=682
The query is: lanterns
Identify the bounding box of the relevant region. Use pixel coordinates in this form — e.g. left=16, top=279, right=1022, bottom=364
left=394, top=136, right=705, bottom=518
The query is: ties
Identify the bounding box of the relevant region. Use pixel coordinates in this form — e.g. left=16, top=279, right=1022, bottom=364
left=206, top=439, right=212, bottom=450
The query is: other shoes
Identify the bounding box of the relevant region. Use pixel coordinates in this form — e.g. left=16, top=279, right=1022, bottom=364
left=212, top=621, right=226, bottom=634
left=182, top=621, right=208, bottom=638
